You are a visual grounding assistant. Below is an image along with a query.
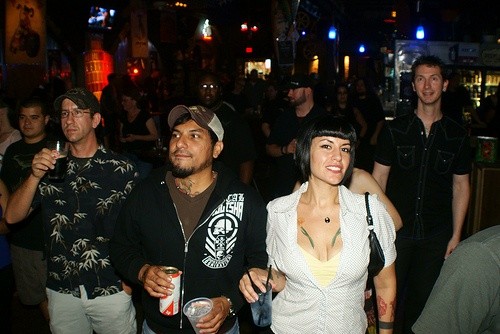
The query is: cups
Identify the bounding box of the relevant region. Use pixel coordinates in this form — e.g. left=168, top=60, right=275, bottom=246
left=49, top=140, right=70, bottom=180
left=183, top=297, right=213, bottom=334
left=250, top=281, right=274, bottom=327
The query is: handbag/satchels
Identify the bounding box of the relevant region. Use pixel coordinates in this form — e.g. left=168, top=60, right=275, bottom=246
left=365, top=192, right=385, bottom=276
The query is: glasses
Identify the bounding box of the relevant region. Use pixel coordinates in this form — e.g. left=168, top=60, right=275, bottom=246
left=60, top=109, right=92, bottom=118
left=200, top=84, right=218, bottom=89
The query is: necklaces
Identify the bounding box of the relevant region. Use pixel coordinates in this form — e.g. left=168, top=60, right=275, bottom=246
left=303, top=191, right=337, bottom=223
left=175, top=171, right=214, bottom=197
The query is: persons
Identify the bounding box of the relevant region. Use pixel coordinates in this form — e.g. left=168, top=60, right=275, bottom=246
left=202, top=19, right=210, bottom=37
left=0, top=54, right=500, bottom=334
left=88, top=6, right=109, bottom=27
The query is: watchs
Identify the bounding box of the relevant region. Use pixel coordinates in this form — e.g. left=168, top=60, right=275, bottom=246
left=219, top=294, right=236, bottom=318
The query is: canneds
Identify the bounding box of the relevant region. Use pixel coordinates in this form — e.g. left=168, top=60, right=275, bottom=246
left=160, top=267, right=180, bottom=316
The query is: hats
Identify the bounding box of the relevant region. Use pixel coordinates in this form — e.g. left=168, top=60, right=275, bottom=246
left=168, top=104, right=225, bottom=141
left=284, top=75, right=313, bottom=88
left=54, top=88, right=98, bottom=112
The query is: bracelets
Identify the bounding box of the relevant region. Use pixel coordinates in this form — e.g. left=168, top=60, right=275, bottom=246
left=280, top=146, right=285, bottom=155
left=285, top=146, right=289, bottom=153
left=379, top=320, right=394, bottom=329
